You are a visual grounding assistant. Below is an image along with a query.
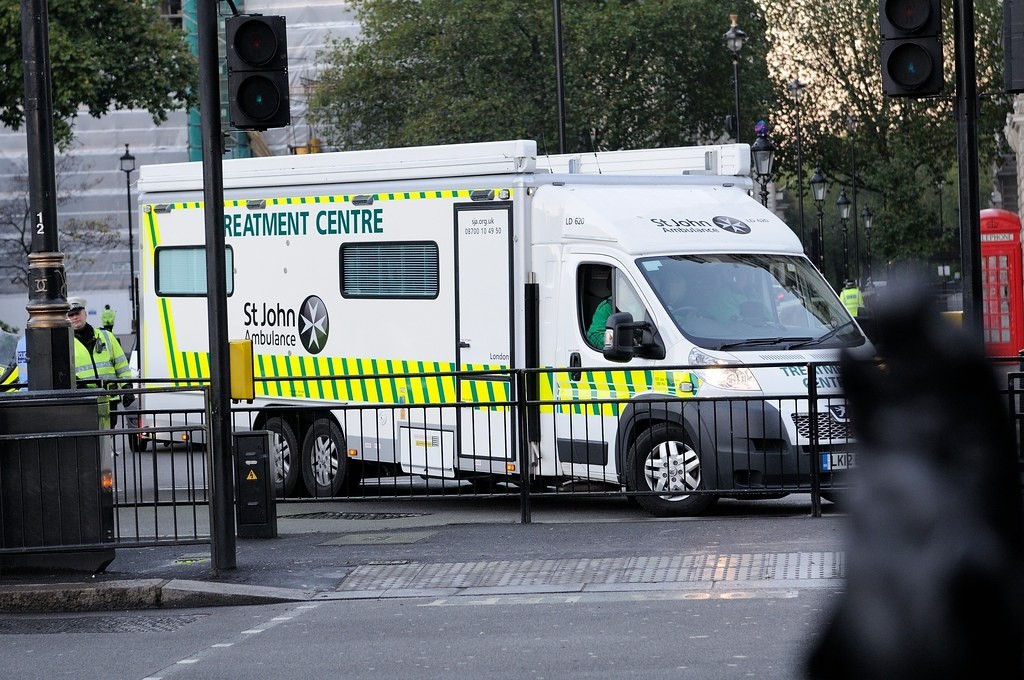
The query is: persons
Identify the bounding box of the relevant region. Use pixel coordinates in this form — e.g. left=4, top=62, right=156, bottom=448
left=588, top=266, right=776, bottom=352
left=67, top=297, right=135, bottom=438
left=840, top=279, right=865, bottom=318
left=101, top=305, right=116, bottom=332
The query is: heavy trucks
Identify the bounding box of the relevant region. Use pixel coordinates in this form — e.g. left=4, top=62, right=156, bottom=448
left=138, top=141, right=890, bottom=517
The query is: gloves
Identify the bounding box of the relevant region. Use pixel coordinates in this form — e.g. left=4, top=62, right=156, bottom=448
left=122, top=384, right=135, bottom=407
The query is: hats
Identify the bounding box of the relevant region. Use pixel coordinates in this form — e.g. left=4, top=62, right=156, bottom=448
left=66, top=297, right=87, bottom=315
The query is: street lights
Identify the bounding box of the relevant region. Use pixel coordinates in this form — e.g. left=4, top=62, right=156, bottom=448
left=861, top=202, right=875, bottom=287
left=935, top=177, right=948, bottom=228
left=788, top=80, right=806, bottom=252
left=808, top=160, right=830, bottom=277
left=119, top=144, right=137, bottom=334
left=835, top=187, right=853, bottom=286
left=846, top=117, right=859, bottom=289
left=723, top=18, right=749, bottom=143
left=749, top=129, right=775, bottom=209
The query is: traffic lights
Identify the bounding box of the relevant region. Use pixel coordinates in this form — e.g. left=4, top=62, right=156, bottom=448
left=225, top=15, right=291, bottom=131
left=879, top=0, right=945, bottom=97
